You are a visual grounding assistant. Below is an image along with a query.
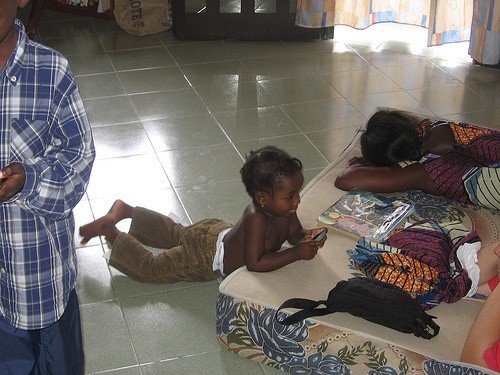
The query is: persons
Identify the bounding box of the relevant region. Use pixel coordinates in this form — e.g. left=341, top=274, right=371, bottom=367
left=80, top=145, right=329, bottom=285
left=1, top=0, right=97, bottom=375
left=334, top=110, right=500, bottom=208
left=460, top=244, right=499, bottom=373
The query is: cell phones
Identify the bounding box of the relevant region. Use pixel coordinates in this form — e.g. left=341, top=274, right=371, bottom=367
left=310, top=229, right=326, bottom=241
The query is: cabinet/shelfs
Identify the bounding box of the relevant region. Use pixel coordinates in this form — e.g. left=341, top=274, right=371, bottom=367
left=172, top=0, right=334, bottom=40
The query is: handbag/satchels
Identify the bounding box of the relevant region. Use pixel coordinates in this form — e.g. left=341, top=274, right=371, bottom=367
left=382, top=218, right=481, bottom=304
left=274, top=277, right=440, bottom=339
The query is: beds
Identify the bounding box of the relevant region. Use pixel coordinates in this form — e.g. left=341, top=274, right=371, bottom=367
left=217, top=106, right=500, bottom=375
